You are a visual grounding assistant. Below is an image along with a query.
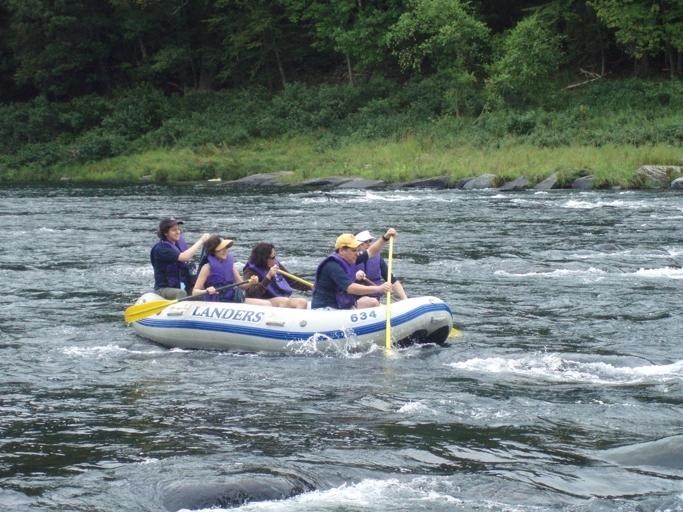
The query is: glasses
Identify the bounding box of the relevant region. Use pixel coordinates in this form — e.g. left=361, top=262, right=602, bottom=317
left=269, top=252, right=277, bottom=260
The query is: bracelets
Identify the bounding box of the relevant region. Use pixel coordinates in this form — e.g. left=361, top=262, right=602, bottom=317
left=383, top=235, right=389, bottom=241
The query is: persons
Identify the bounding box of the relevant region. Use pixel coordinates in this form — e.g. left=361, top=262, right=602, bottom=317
left=191, top=234, right=272, bottom=307
left=353, top=230, right=407, bottom=309
left=150, top=216, right=211, bottom=301
left=243, top=242, right=312, bottom=310
left=310, top=227, right=397, bottom=310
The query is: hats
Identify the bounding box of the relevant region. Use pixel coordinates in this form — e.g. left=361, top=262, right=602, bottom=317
left=159, top=215, right=184, bottom=230
left=211, top=235, right=234, bottom=252
left=335, top=234, right=364, bottom=251
left=354, top=230, right=374, bottom=242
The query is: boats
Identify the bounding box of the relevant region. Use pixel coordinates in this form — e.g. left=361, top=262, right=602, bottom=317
left=130, top=291, right=453, bottom=355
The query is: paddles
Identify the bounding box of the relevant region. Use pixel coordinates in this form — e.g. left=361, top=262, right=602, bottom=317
left=123, top=275, right=257, bottom=323
left=386, top=232, right=392, bottom=350
